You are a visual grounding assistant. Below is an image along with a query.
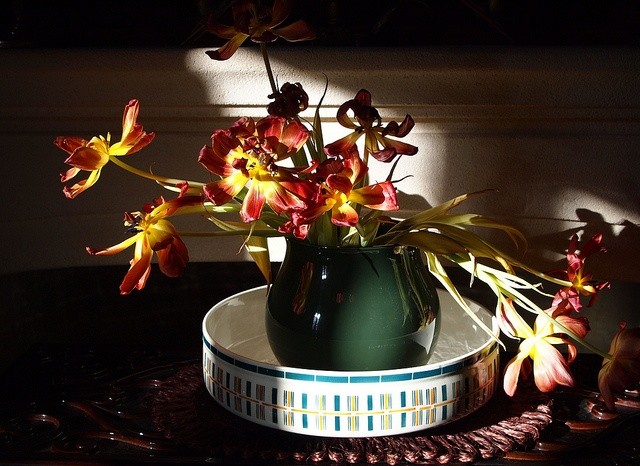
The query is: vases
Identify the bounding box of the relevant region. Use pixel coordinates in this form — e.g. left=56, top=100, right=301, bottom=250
left=238, top=235, right=441, bottom=371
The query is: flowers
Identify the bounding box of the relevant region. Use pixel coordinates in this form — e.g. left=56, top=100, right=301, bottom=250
left=55, top=2, right=611, bottom=396
